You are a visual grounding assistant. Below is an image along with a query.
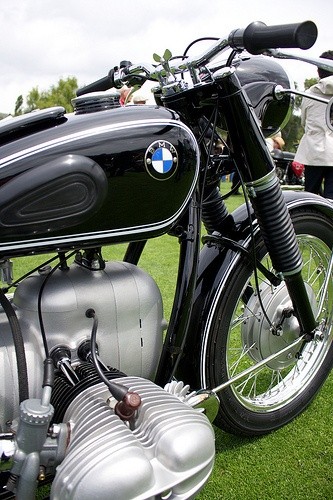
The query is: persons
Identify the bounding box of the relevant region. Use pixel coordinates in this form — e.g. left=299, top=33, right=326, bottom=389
left=293, top=51, right=333, bottom=200
left=120, top=84, right=285, bottom=195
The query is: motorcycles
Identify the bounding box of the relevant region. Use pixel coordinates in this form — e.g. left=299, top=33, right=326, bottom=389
left=0, top=17, right=333, bottom=496
left=270, top=148, right=303, bottom=187
